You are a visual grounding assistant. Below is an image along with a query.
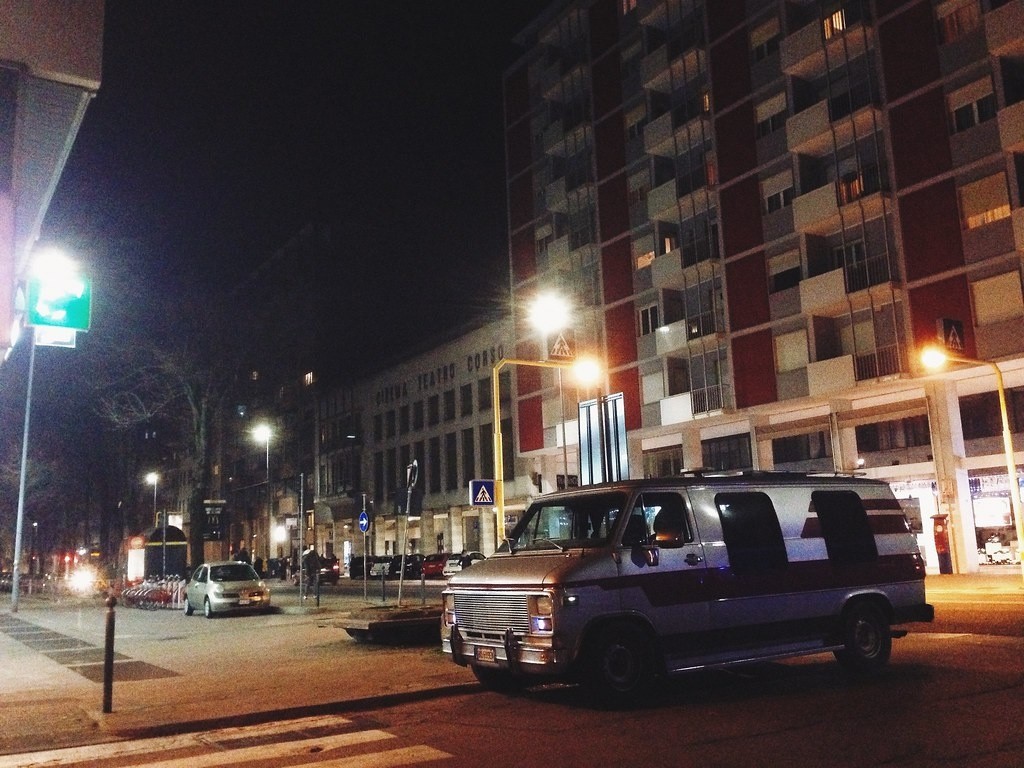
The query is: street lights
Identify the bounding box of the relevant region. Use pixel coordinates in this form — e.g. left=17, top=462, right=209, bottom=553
left=253, top=425, right=274, bottom=570
left=922, top=347, right=1023, bottom=566
left=147, top=471, right=159, bottom=522
left=492, top=357, right=604, bottom=546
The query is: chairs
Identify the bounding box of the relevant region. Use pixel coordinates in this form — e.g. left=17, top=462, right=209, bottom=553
left=606, top=514, right=649, bottom=545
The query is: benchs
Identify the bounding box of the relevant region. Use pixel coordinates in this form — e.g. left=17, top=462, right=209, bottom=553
left=316, top=618, right=438, bottom=642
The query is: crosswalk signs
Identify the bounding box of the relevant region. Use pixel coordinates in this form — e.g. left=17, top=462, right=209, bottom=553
left=469, top=479, right=494, bottom=507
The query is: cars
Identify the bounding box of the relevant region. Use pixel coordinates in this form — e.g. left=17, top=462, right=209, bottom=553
left=291, top=559, right=339, bottom=587
left=368, top=555, right=394, bottom=577
left=442, top=551, right=487, bottom=576
left=182, top=561, right=270, bottom=618
left=419, top=552, right=450, bottom=576
left=349, top=555, right=378, bottom=579
left=390, top=553, right=428, bottom=578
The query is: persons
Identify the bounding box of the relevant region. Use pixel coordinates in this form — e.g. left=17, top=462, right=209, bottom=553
left=229, top=547, right=286, bottom=584
left=304, top=550, right=321, bottom=599
left=457, top=549, right=473, bottom=570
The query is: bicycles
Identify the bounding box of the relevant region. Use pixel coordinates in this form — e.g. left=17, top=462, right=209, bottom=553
left=120, top=573, right=185, bottom=612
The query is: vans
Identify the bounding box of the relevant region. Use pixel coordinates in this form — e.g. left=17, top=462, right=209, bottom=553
left=438, top=466, right=933, bottom=712
